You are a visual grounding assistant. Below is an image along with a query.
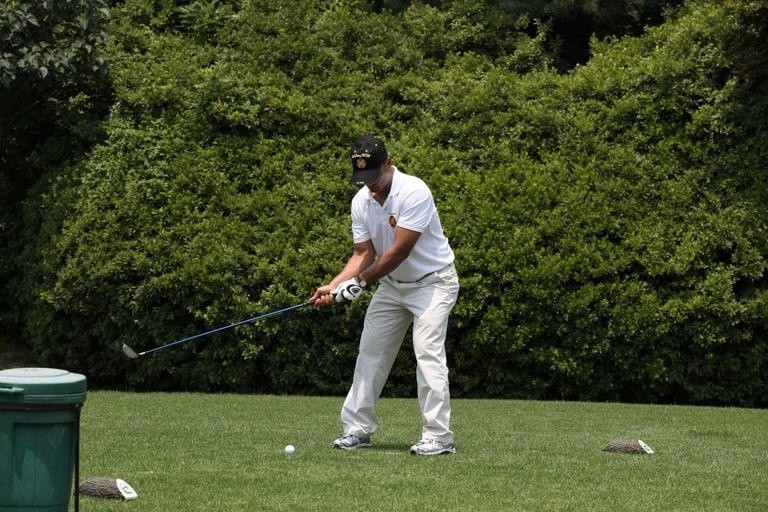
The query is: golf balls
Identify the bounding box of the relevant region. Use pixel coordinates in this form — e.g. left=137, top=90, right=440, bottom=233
left=284, top=445, right=294, bottom=453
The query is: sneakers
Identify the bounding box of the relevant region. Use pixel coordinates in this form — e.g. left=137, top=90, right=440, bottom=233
left=411, top=438, right=457, bottom=456
left=328, top=433, right=371, bottom=451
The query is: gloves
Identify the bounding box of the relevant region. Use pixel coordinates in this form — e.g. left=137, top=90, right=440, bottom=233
left=329, top=274, right=364, bottom=306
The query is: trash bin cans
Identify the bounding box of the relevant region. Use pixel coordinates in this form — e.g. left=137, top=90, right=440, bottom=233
left=0, top=367, right=87, bottom=512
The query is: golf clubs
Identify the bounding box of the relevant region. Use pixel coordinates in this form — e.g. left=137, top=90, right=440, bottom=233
left=122, top=295, right=337, bottom=359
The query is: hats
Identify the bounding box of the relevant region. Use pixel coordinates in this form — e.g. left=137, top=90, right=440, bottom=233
left=349, top=137, right=388, bottom=186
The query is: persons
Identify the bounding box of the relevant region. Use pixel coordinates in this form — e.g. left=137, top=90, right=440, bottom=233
left=308, top=135, right=464, bottom=457
